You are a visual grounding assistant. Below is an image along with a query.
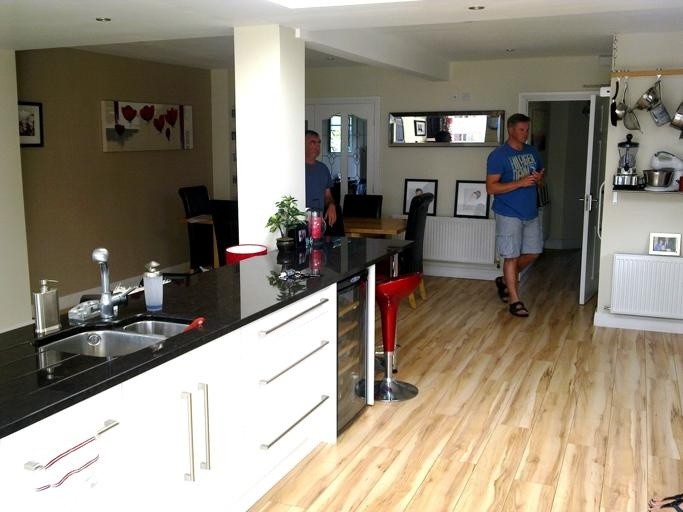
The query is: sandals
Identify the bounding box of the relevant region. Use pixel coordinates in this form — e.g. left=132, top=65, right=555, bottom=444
left=495, top=276, right=510, bottom=303
left=510, top=301, right=530, bottom=317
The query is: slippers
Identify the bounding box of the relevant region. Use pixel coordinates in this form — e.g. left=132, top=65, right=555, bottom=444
left=647, top=493, right=683, bottom=512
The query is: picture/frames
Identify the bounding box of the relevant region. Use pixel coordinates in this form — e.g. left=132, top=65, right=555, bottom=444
left=403, top=179, right=437, bottom=216
left=414, top=120, right=426, bottom=136
left=649, top=232, right=681, bottom=256
left=454, top=180, right=490, bottom=219
left=18, top=100, right=44, bottom=148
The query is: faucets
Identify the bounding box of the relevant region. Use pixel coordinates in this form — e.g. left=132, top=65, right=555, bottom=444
left=90, top=246, right=119, bottom=320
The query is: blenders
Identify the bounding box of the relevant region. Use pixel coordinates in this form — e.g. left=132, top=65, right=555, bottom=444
left=613, top=134, right=640, bottom=187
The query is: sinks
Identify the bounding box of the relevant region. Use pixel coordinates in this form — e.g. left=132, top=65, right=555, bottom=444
left=41, top=330, right=162, bottom=358
left=123, top=320, right=193, bottom=337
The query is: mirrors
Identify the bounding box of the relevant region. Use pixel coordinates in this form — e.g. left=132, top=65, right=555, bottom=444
left=387, top=110, right=504, bottom=146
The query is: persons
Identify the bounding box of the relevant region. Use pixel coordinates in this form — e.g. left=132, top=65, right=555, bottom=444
left=415, top=188, right=423, bottom=197
left=646, top=493, right=683, bottom=512
left=304, top=129, right=336, bottom=232
left=466, top=190, right=480, bottom=212
left=485, top=113, right=548, bottom=318
left=654, top=239, right=664, bottom=250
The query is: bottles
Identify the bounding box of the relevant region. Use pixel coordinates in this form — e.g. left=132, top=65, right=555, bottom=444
left=309, top=209, right=321, bottom=239
left=309, top=250, right=321, bottom=277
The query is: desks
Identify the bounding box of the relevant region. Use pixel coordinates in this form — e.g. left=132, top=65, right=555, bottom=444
left=179, top=214, right=408, bottom=268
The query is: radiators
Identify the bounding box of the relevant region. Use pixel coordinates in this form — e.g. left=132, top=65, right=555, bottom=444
left=604, top=254, right=683, bottom=320
left=423, top=216, right=501, bottom=269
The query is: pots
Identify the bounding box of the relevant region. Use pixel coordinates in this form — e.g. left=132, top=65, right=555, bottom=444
left=642, top=169, right=674, bottom=188
left=610, top=75, right=683, bottom=139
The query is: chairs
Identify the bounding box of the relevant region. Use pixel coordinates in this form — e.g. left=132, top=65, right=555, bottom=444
left=178, top=185, right=223, bottom=270
left=211, top=200, right=238, bottom=268
left=375, top=193, right=433, bottom=309
left=343, top=195, right=382, bottom=219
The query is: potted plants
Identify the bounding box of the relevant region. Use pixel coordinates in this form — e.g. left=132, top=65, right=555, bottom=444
left=265, top=195, right=308, bottom=264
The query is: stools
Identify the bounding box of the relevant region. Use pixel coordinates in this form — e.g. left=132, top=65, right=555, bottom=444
left=375, top=272, right=422, bottom=401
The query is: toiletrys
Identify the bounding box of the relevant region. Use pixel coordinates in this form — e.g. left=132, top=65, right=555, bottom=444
left=36, top=347, right=65, bottom=381
left=32, top=279, right=62, bottom=334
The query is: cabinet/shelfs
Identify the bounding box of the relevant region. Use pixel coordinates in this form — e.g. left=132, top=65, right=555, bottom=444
left=340, top=277, right=368, bottom=436
left=262, top=279, right=339, bottom=496
left=144, top=319, right=262, bottom=511
left=0, top=378, right=162, bottom=512
